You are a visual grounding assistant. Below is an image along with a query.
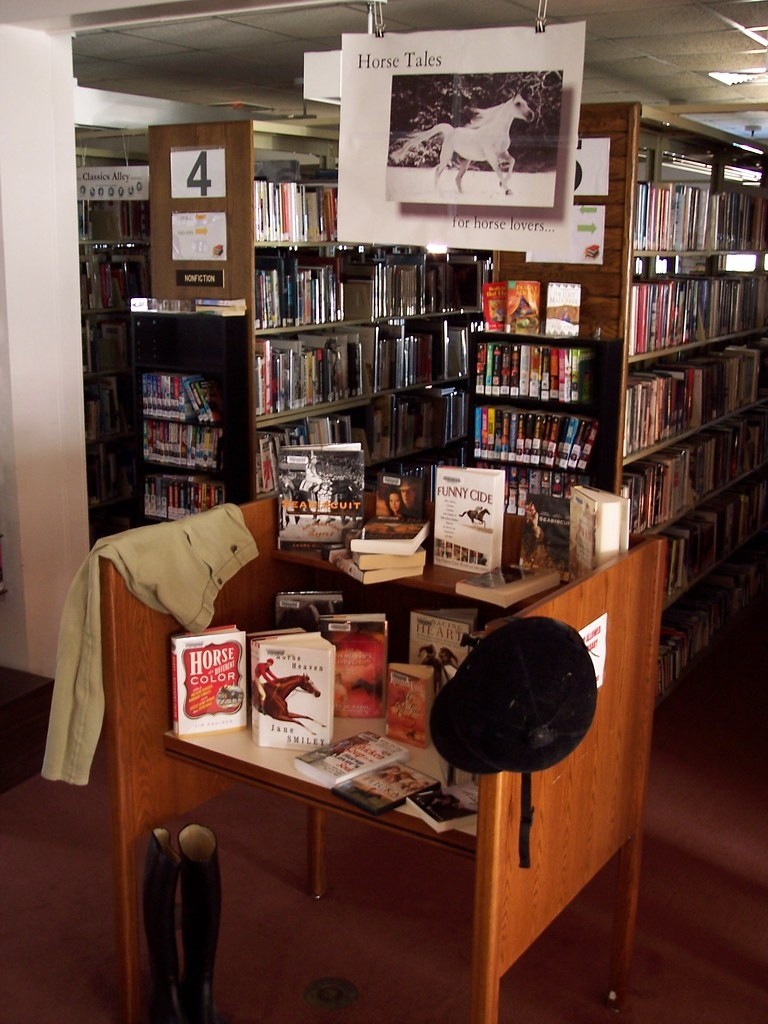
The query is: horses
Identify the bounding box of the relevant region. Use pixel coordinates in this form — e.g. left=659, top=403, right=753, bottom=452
left=251, top=673, right=328, bottom=735
left=390, top=87, right=535, bottom=196
left=459, top=508, right=490, bottom=524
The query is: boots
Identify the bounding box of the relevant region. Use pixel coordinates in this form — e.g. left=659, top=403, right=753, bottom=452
left=142, top=827, right=182, bottom=1024
left=177, top=822, right=227, bottom=1024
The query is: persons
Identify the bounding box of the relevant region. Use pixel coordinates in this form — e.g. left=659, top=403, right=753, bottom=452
left=388, top=489, right=403, bottom=517
left=399, top=481, right=419, bottom=518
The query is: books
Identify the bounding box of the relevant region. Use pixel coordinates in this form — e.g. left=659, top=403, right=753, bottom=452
left=81, top=169, right=768, bottom=833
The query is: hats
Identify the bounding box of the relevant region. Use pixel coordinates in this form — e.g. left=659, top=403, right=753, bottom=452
left=429, top=615, right=596, bottom=775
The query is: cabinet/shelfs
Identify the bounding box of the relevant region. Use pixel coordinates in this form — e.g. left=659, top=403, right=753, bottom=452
left=463, top=328, right=625, bottom=519
left=488, top=100, right=768, bottom=709
left=76, top=143, right=145, bottom=552
left=101, top=495, right=666, bottom=1023
left=146, top=120, right=495, bottom=530
